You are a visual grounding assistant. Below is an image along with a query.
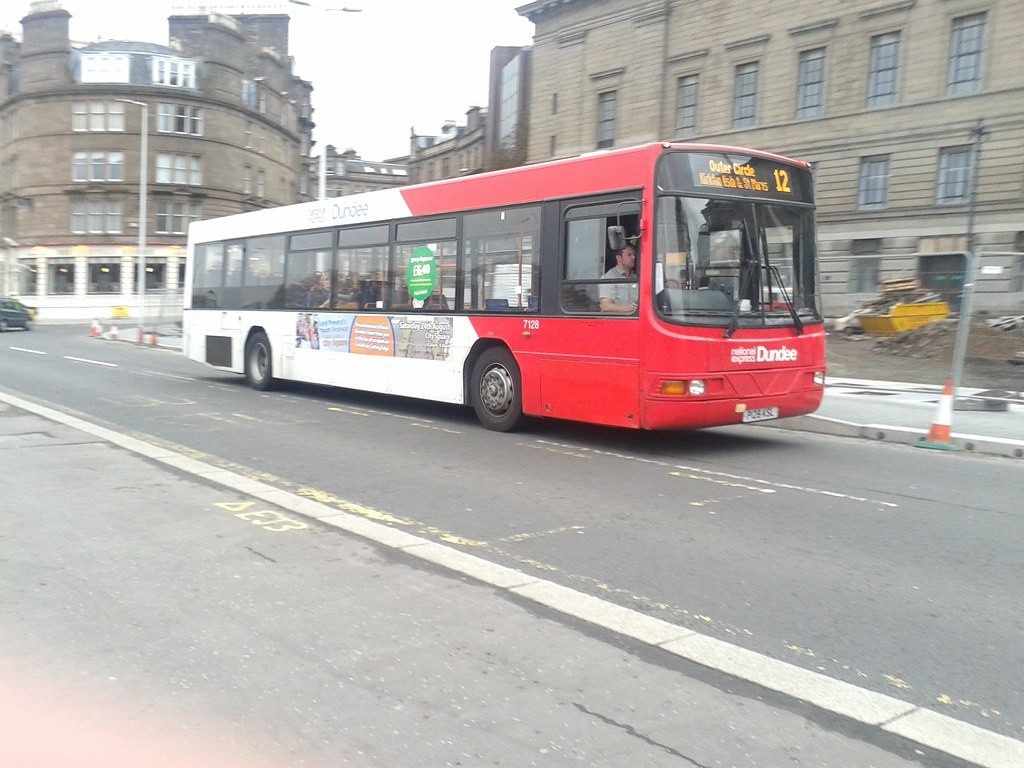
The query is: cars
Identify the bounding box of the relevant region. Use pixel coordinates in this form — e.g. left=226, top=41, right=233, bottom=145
left=0, top=299, right=35, bottom=332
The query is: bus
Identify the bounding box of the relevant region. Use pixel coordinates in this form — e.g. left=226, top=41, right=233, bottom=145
left=181, top=142, right=828, bottom=432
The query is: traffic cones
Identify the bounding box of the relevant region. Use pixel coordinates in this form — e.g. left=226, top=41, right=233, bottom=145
left=915, top=380, right=959, bottom=451
left=88, top=319, right=158, bottom=348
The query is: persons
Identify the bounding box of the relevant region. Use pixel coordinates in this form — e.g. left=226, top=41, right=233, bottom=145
left=597, top=243, right=638, bottom=313
left=335, top=280, right=381, bottom=309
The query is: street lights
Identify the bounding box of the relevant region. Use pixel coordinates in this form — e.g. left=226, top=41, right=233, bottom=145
left=288, top=0, right=363, bottom=273
left=116, top=99, right=148, bottom=322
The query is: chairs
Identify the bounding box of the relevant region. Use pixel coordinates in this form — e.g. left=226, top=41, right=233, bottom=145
left=425, top=293, right=539, bottom=312
